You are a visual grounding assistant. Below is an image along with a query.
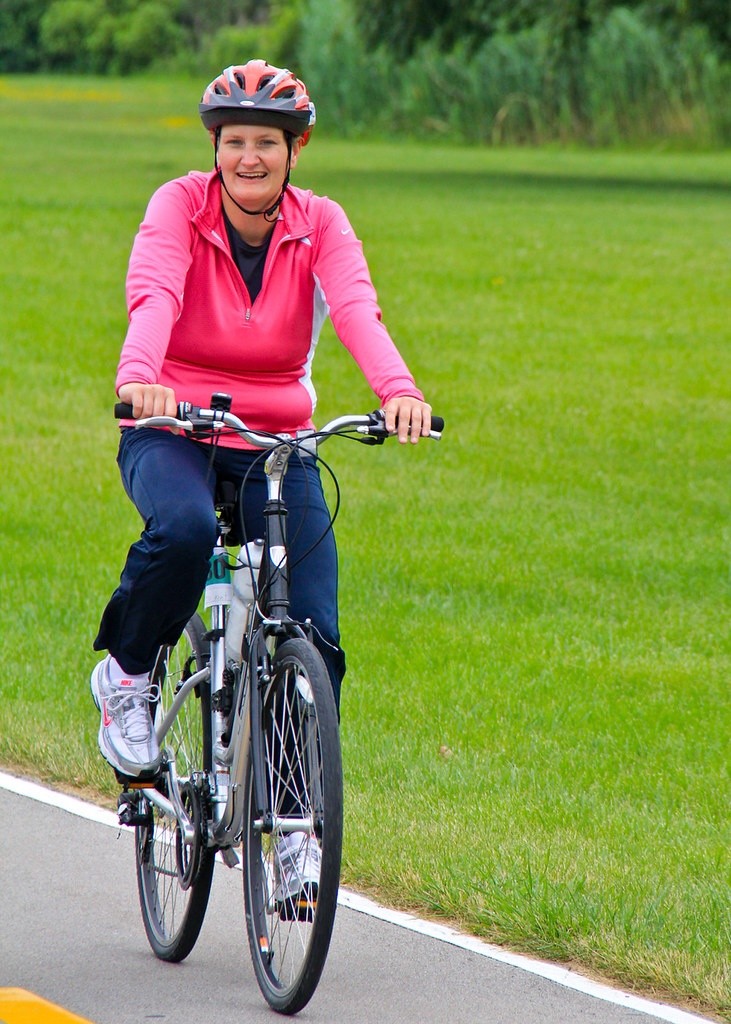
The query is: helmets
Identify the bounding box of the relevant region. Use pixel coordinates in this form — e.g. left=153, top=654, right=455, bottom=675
left=198, top=59, right=317, bottom=147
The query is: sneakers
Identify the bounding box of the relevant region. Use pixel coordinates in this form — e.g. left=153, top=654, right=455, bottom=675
left=273, top=831, right=323, bottom=903
left=90, top=652, right=163, bottom=781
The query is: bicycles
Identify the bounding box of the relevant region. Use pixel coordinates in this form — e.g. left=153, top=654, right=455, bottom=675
left=112, top=388, right=449, bottom=1016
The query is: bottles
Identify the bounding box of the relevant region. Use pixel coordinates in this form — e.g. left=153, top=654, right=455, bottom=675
left=225, top=537, right=274, bottom=661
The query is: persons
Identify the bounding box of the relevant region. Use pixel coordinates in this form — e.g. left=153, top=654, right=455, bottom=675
left=87, top=59, right=435, bottom=926
